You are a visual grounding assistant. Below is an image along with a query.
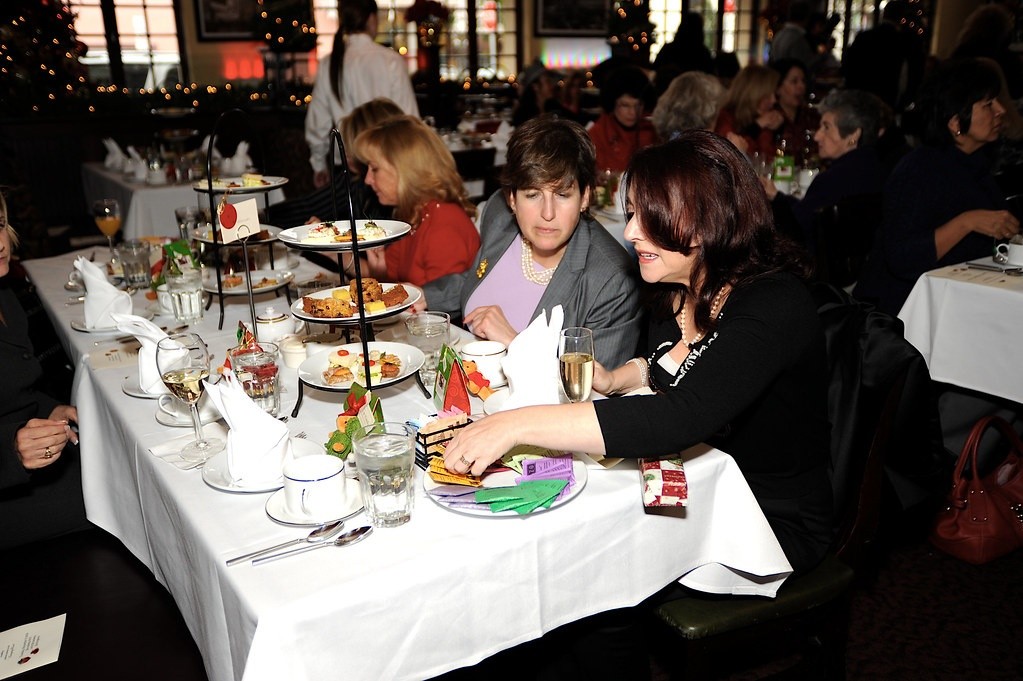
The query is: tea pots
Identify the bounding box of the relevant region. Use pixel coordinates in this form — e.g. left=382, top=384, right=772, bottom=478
left=290, top=283, right=422, bottom=323
left=297, top=341, right=427, bottom=391
left=243, top=307, right=305, bottom=351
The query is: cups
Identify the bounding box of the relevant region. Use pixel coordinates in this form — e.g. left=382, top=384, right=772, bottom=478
left=163, top=267, right=205, bottom=327
left=117, top=238, right=153, bottom=289
left=256, top=245, right=288, bottom=271
left=175, top=205, right=207, bottom=251
left=460, top=341, right=507, bottom=388
left=106, top=154, right=166, bottom=185
left=996, top=242, right=1023, bottom=266
left=558, top=327, right=595, bottom=403
left=158, top=393, right=191, bottom=422
left=403, top=311, right=450, bottom=387
left=229, top=342, right=281, bottom=419
left=282, top=454, right=347, bottom=517
left=156, top=284, right=175, bottom=314
left=351, top=421, right=418, bottom=529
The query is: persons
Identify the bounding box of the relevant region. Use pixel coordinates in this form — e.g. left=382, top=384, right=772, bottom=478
left=304, top=115, right=481, bottom=287
left=0, top=504, right=208, bottom=681
left=444, top=131, right=829, bottom=681
left=306, top=0, right=420, bottom=188
left=404, top=114, right=640, bottom=371
left=0, top=195, right=84, bottom=512
left=511, top=0, right=1023, bottom=465
left=258, top=97, right=407, bottom=278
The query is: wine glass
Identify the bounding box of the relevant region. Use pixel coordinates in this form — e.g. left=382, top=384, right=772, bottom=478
left=155, top=332, right=226, bottom=461
left=93, top=198, right=121, bottom=262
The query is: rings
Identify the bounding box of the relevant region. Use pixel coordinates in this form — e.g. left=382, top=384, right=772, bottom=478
left=45, top=447, right=52, bottom=459
left=460, top=456, right=472, bottom=466
left=1003, top=231, right=1010, bottom=236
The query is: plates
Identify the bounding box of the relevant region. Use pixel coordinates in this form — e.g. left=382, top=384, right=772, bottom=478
left=265, top=475, right=366, bottom=528
left=288, top=256, right=300, bottom=269
left=276, top=219, right=412, bottom=248
left=202, top=269, right=295, bottom=294
left=70, top=307, right=153, bottom=332
left=121, top=377, right=158, bottom=399
left=189, top=223, right=285, bottom=246
left=201, top=437, right=329, bottom=494
left=192, top=176, right=289, bottom=193
left=422, top=450, right=588, bottom=517
left=155, top=410, right=194, bottom=428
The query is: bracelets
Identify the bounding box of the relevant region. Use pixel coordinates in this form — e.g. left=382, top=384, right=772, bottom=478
left=626, top=359, right=646, bottom=388
left=345, top=253, right=354, bottom=271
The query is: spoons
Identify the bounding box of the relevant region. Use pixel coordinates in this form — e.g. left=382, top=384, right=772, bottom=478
left=252, top=525, right=372, bottom=566
left=226, top=521, right=345, bottom=567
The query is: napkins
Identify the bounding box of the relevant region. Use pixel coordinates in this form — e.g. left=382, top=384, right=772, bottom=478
left=72, top=255, right=132, bottom=328
left=501, top=305, right=565, bottom=407
left=104, top=135, right=253, bottom=185
left=109, top=312, right=191, bottom=393
left=200, top=374, right=289, bottom=483
left=448, top=120, right=515, bottom=165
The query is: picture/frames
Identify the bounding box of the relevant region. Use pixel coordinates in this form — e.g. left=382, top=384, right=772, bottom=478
left=192, top=0, right=261, bottom=42
left=535, top=0, right=612, bottom=37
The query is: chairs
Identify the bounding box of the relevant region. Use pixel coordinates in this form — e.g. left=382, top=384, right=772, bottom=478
left=656, top=282, right=913, bottom=681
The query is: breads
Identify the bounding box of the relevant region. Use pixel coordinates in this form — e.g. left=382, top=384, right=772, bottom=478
left=253, top=278, right=277, bottom=288
left=221, top=275, right=243, bottom=288
left=208, top=229, right=271, bottom=241
left=301, top=277, right=408, bottom=319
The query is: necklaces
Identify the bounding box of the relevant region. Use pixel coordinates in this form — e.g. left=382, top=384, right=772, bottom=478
left=522, top=235, right=558, bottom=286
left=681, top=287, right=725, bottom=346
left=408, top=203, right=442, bottom=235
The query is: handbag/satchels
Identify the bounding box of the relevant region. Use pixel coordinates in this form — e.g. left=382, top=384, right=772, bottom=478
left=929, top=416, right=1023, bottom=561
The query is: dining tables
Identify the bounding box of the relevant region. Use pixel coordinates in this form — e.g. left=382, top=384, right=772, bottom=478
left=83, top=152, right=286, bottom=242
left=21, top=242, right=794, bottom=681
left=897, top=252, right=1023, bottom=405
left=475, top=168, right=820, bottom=259
left=439, top=130, right=496, bottom=180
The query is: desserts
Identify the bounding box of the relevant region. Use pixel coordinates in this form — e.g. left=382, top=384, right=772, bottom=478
left=308, top=221, right=386, bottom=243
left=198, top=176, right=228, bottom=189
left=322, top=349, right=400, bottom=385
left=239, top=168, right=264, bottom=187
